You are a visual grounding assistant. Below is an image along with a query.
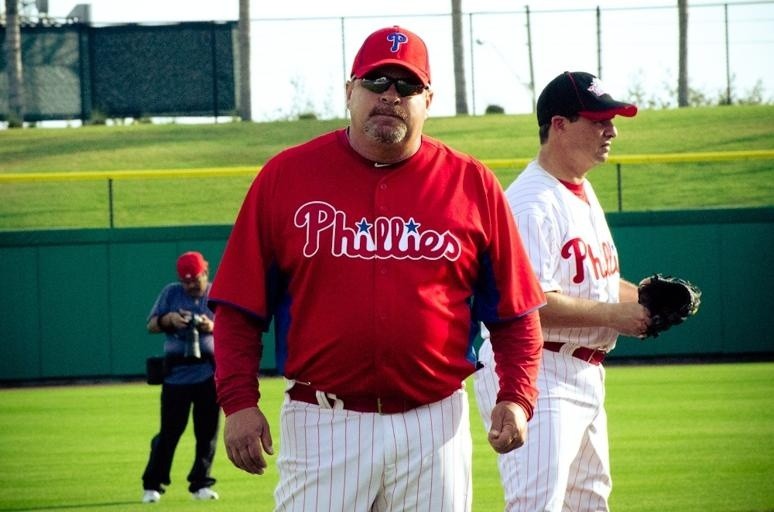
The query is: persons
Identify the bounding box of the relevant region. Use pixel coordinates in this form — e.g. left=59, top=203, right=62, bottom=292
left=206, top=23, right=546, bottom=512
left=471, top=70, right=700, bottom=512
left=141, top=252, right=220, bottom=502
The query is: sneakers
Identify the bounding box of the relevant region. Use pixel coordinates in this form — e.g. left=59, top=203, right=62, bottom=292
left=191, top=488, right=219, bottom=500
left=142, top=490, right=162, bottom=504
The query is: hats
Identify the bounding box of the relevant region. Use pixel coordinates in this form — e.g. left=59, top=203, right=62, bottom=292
left=355, top=25, right=432, bottom=88
left=537, top=71, right=637, bottom=126
left=176, top=253, right=209, bottom=280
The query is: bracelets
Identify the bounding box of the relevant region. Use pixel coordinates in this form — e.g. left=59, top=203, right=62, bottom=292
left=156, top=313, right=166, bottom=331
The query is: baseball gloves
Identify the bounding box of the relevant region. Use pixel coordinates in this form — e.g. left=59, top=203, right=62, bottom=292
left=637, top=272, right=702, bottom=341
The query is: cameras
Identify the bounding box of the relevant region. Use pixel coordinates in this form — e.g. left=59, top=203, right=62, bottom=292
left=181, top=314, right=204, bottom=362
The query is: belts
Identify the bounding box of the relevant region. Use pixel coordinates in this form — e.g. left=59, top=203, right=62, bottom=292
left=289, top=382, right=418, bottom=414
left=542, top=341, right=607, bottom=365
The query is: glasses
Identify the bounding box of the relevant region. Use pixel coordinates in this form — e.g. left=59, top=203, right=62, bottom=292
left=352, top=73, right=425, bottom=97
